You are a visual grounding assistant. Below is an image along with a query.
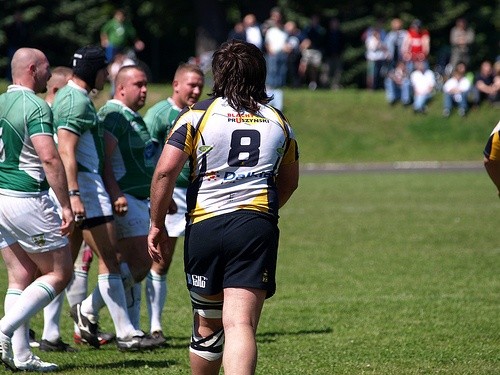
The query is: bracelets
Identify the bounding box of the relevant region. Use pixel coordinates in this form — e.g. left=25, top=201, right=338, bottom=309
left=68, top=190, right=80, bottom=196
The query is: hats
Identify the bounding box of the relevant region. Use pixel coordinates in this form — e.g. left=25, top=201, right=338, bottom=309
left=72, top=45, right=110, bottom=88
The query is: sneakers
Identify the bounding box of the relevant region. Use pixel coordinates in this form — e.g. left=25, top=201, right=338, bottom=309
left=70, top=303, right=100, bottom=349
left=14, top=353, right=59, bottom=371
left=73, top=331, right=116, bottom=345
left=117, top=331, right=166, bottom=352
left=39, top=337, right=78, bottom=352
left=0, top=329, right=18, bottom=371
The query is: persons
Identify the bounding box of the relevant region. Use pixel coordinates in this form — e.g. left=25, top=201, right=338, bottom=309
left=192, top=8, right=500, bottom=196
left=0, top=10, right=206, bottom=371
left=148, top=39, right=302, bottom=375
